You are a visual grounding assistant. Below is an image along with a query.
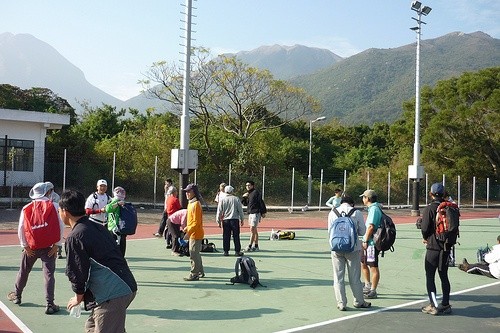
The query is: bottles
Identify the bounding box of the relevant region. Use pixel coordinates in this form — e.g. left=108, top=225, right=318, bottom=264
left=70, top=297, right=81, bottom=319
left=270, top=229, right=275, bottom=241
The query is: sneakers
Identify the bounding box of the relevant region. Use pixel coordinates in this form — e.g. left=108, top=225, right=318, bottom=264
left=422, top=304, right=438, bottom=314
left=437, top=303, right=451, bottom=313
left=362, top=288, right=371, bottom=296
left=45, top=305, right=59, bottom=314
left=365, top=291, right=377, bottom=299
left=7, top=292, right=21, bottom=304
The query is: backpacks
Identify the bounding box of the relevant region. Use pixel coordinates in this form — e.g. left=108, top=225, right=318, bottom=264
left=178, top=238, right=190, bottom=256
left=372, top=206, right=396, bottom=251
left=230, top=257, right=259, bottom=289
left=329, top=208, right=357, bottom=252
left=113, top=203, right=137, bottom=236
left=200, top=239, right=213, bottom=252
left=477, top=248, right=485, bottom=262
left=260, top=199, right=266, bottom=214
left=430, top=201, right=459, bottom=241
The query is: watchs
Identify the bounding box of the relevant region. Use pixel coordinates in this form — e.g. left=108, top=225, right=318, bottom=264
left=364, top=240, right=368, bottom=243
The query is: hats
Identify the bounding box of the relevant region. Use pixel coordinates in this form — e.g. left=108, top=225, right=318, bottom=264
left=359, top=190, right=377, bottom=197
left=97, top=179, right=107, bottom=186
left=167, top=186, right=176, bottom=194
left=45, top=182, right=54, bottom=190
left=431, top=182, right=445, bottom=195
left=225, top=186, right=234, bottom=192
left=29, top=183, right=48, bottom=199
left=183, top=184, right=198, bottom=191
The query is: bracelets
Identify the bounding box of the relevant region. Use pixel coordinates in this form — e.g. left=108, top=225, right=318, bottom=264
left=117, top=202, right=120, bottom=206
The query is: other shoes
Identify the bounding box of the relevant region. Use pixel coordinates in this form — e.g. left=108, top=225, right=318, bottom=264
left=166, top=246, right=172, bottom=249
left=224, top=251, right=230, bottom=255
left=171, top=252, right=180, bottom=256
left=57, top=254, right=62, bottom=259
left=199, top=272, right=204, bottom=278
left=463, top=258, right=470, bottom=268
left=354, top=301, right=371, bottom=308
left=337, top=303, right=346, bottom=311
left=243, top=248, right=253, bottom=251
left=235, top=252, right=244, bottom=256
left=184, top=274, right=199, bottom=281
left=251, top=248, right=259, bottom=251
left=458, top=264, right=469, bottom=272
left=153, top=233, right=162, bottom=237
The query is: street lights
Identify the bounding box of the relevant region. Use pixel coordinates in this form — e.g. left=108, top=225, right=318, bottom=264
left=407, top=1, right=432, bottom=216
left=308, top=116, right=327, bottom=205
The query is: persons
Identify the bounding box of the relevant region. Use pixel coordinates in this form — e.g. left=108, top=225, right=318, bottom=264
left=328, top=197, right=371, bottom=310
left=8, top=183, right=64, bottom=315
left=84, top=179, right=113, bottom=226
left=45, top=182, right=64, bottom=259
left=56, top=191, right=138, bottom=333
left=459, top=235, right=500, bottom=280
left=215, top=186, right=245, bottom=256
left=215, top=183, right=227, bottom=206
left=421, top=183, right=460, bottom=315
left=183, top=183, right=205, bottom=281
left=104, top=187, right=138, bottom=256
left=166, top=208, right=196, bottom=256
left=326, top=190, right=344, bottom=212
left=243, top=181, right=267, bottom=252
left=358, top=190, right=383, bottom=299
left=153, top=178, right=187, bottom=249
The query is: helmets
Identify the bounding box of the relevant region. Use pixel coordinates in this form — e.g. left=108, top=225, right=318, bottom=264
left=114, top=186, right=126, bottom=199
